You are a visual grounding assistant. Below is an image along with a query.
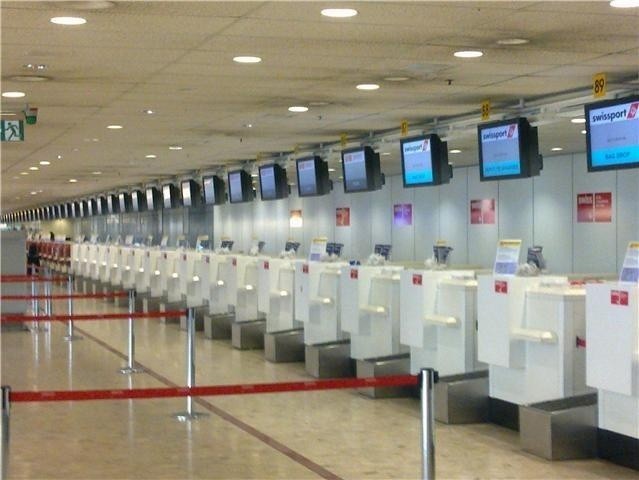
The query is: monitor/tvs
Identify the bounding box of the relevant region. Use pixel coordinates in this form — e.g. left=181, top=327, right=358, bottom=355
left=400, top=134, right=452, bottom=187
left=0, top=183, right=179, bottom=223
left=584, top=95, right=639, bottom=172
left=296, top=156, right=333, bottom=197
left=477, top=117, right=543, bottom=181
left=228, top=169, right=254, bottom=203
left=181, top=179, right=199, bottom=208
left=341, top=146, right=384, bottom=193
left=258, top=163, right=291, bottom=200
left=202, top=176, right=224, bottom=206
